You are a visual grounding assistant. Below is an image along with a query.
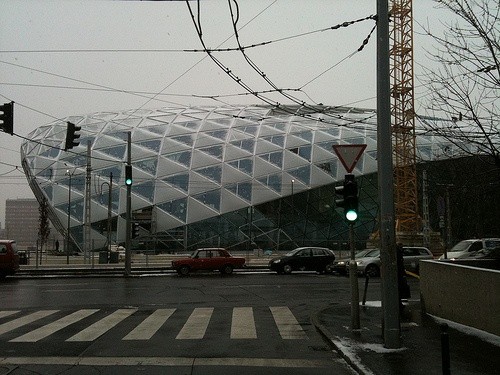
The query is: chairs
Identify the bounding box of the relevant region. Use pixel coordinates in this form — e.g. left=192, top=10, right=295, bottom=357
left=2, top=248, right=7, bottom=253
left=403, top=251, right=410, bottom=256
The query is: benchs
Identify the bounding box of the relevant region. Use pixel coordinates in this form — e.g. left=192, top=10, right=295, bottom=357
left=27, top=247, right=37, bottom=253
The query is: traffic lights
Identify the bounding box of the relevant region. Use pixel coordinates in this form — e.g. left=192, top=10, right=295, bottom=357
left=64, top=120, right=82, bottom=152
left=131, top=222, right=140, bottom=239
left=124, top=165, right=133, bottom=188
left=0, top=100, right=15, bottom=136
left=342, top=178, right=360, bottom=225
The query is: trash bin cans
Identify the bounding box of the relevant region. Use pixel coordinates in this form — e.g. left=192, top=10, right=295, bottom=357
left=110, top=252, right=119, bottom=263
left=99, top=251, right=108, bottom=264
left=18, top=251, right=26, bottom=264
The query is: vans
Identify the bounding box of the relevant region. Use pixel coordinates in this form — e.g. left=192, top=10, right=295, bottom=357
left=439, top=238, right=500, bottom=260
left=334, top=246, right=436, bottom=276
left=0, top=240, right=20, bottom=278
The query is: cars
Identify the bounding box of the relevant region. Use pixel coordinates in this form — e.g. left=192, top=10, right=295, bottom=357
left=268, top=247, right=336, bottom=274
left=171, top=248, right=246, bottom=274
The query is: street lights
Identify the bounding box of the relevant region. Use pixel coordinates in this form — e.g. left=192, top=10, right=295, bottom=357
left=63, top=162, right=83, bottom=264
